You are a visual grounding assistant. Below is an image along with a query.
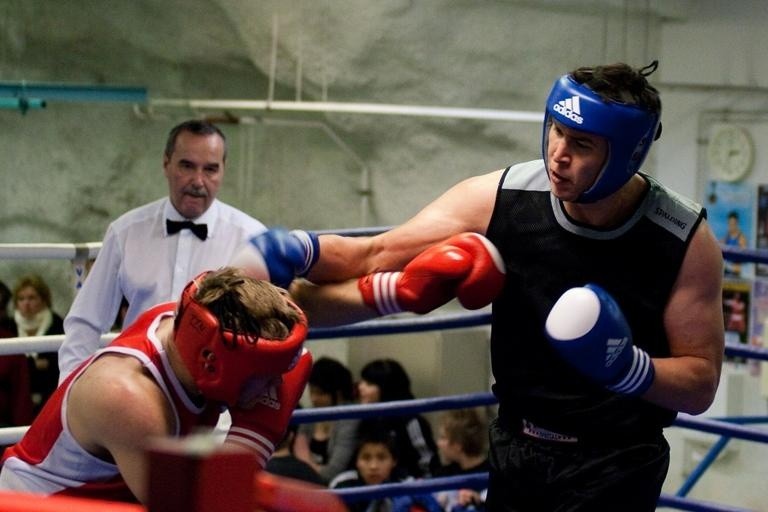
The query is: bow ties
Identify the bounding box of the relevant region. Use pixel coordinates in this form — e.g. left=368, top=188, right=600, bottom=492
left=166, top=218, right=207, bottom=240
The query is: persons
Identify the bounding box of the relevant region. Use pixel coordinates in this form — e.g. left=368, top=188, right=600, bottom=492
left=0, top=230, right=508, bottom=512
left=265, top=353, right=492, bottom=512
left=228, top=59, right=727, bottom=511
left=8, top=273, right=65, bottom=415
left=720, top=212, right=747, bottom=279
left=723, top=292, right=747, bottom=344
left=0, top=279, right=33, bottom=430
left=55, top=120, right=268, bottom=387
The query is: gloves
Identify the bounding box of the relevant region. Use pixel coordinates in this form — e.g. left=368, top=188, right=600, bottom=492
left=357, top=232, right=504, bottom=315
left=546, top=284, right=654, bottom=398
left=228, top=229, right=319, bottom=290
left=227, top=348, right=312, bottom=459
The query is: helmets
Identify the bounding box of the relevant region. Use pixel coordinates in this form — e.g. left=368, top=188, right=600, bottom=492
left=542, top=73, right=662, bottom=204
left=172, top=270, right=307, bottom=406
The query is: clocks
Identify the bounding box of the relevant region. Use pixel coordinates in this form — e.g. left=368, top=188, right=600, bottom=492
left=707, top=124, right=756, bottom=183
